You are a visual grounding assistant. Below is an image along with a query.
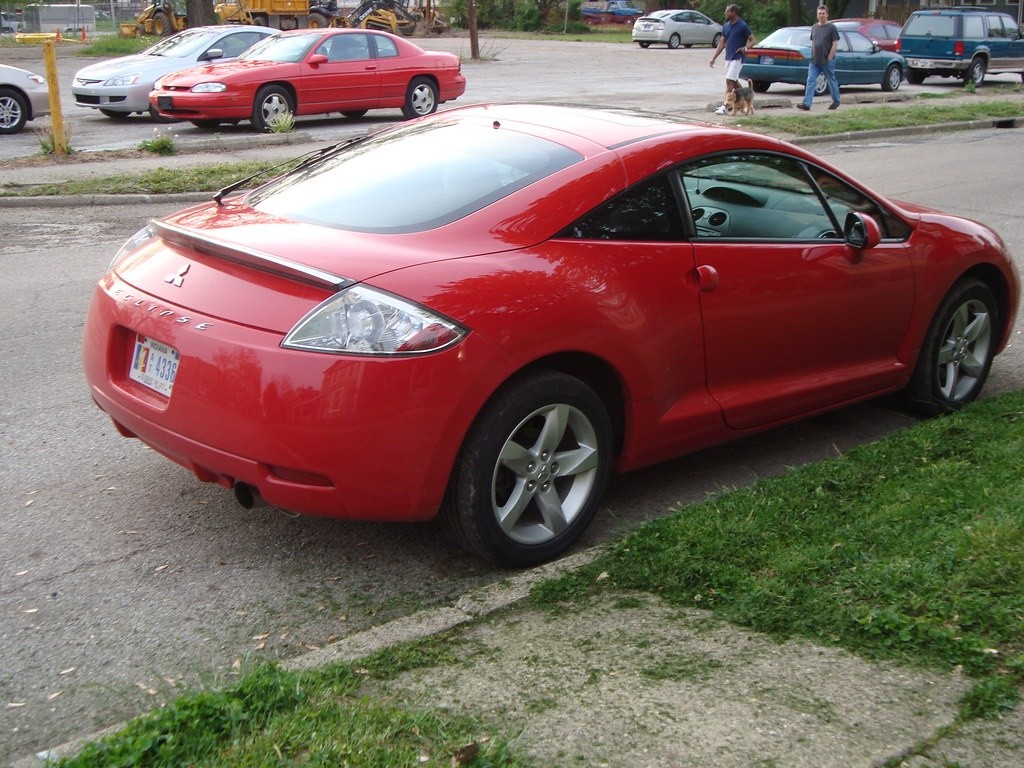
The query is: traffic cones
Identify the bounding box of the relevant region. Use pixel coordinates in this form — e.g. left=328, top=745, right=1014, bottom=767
left=56, top=29, right=62, bottom=43
left=80, top=26, right=87, bottom=42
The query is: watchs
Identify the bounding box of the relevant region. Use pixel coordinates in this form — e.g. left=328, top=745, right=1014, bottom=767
left=744, top=46, right=748, bottom=52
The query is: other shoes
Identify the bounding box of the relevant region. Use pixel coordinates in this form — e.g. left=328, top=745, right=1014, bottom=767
left=796, top=103, right=810, bottom=111
left=827, top=102, right=840, bottom=110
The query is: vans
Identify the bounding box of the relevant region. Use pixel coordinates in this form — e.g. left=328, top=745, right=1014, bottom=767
left=894, top=5, right=1024, bottom=87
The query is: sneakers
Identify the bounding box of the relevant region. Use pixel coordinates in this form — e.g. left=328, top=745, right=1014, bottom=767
left=715, top=105, right=730, bottom=115
left=741, top=105, right=753, bottom=113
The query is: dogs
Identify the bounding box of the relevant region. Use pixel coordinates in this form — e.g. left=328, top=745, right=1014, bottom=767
left=725, top=79, right=755, bottom=116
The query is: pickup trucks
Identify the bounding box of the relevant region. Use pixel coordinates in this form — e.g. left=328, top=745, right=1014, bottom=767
left=576, top=0, right=645, bottom=27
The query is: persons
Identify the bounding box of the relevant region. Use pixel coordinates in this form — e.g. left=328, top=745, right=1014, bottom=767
left=710, top=5, right=755, bottom=114
left=796, top=5, right=840, bottom=109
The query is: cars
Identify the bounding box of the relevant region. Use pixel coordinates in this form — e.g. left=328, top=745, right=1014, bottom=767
left=0, top=11, right=26, bottom=36
left=826, top=16, right=906, bottom=52
left=631, top=7, right=724, bottom=51
left=82, top=100, right=1022, bottom=570
left=734, top=24, right=910, bottom=93
left=0, top=63, right=54, bottom=135
left=149, top=25, right=468, bottom=133
left=69, top=24, right=288, bottom=123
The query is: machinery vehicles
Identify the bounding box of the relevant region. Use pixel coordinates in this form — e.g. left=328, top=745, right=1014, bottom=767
left=118, top=0, right=188, bottom=39
left=213, top=0, right=339, bottom=32
left=330, top=0, right=449, bottom=36
